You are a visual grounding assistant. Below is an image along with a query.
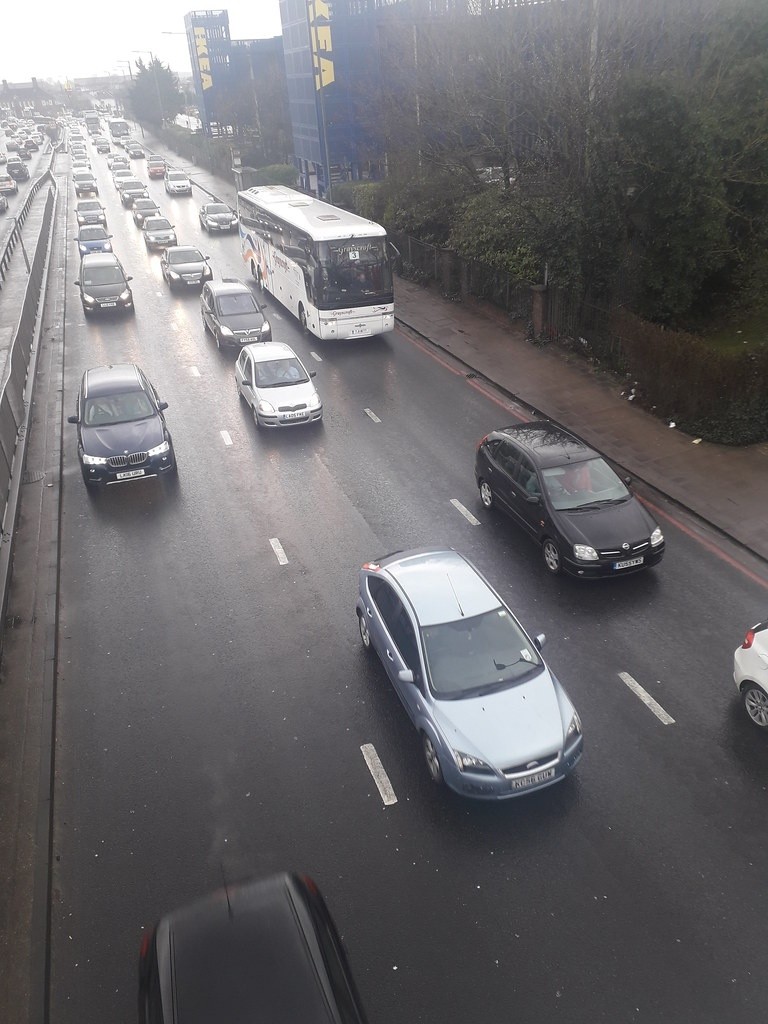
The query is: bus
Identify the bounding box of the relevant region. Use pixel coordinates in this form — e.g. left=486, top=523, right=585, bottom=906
left=237, top=184, right=405, bottom=341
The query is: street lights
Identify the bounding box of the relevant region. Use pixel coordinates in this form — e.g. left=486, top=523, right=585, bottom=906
left=132, top=50, right=165, bottom=120
left=160, top=31, right=198, bottom=82
left=116, top=59, right=132, bottom=79
left=117, top=65, right=127, bottom=85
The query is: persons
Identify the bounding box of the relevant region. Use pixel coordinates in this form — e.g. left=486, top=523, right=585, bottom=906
left=354, top=274, right=370, bottom=288
left=88, top=399, right=119, bottom=422
left=277, top=361, right=300, bottom=379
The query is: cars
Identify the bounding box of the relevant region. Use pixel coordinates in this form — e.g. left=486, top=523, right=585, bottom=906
left=161, top=244, right=213, bottom=291
left=137, top=865, right=366, bottom=1024
left=199, top=202, right=239, bottom=233
left=133, top=198, right=162, bottom=229
left=200, top=277, right=272, bottom=352
left=356, top=544, right=585, bottom=802
left=146, top=162, right=167, bottom=178
left=73, top=198, right=108, bottom=229
left=73, top=252, right=135, bottom=319
left=66, top=362, right=175, bottom=493
left=142, top=215, right=178, bottom=251
left=0, top=109, right=146, bottom=212
left=234, top=342, right=324, bottom=430
left=165, top=170, right=193, bottom=196
left=146, top=155, right=168, bottom=164
left=474, top=419, right=667, bottom=585
left=732, top=620, right=768, bottom=731
left=117, top=179, right=149, bottom=207
left=72, top=225, right=114, bottom=258
